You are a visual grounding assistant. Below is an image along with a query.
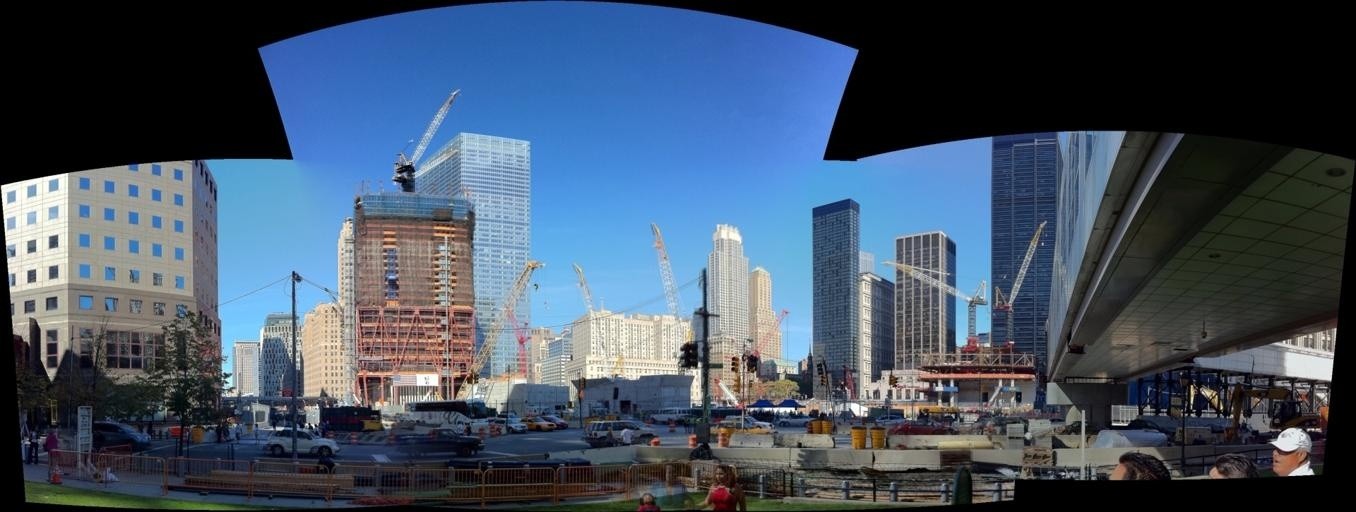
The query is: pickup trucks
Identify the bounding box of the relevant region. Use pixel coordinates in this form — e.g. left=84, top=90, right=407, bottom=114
left=397, top=428, right=485, bottom=458
left=1106, top=418, right=1175, bottom=446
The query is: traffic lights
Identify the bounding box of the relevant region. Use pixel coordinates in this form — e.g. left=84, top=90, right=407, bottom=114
left=889, top=375, right=898, bottom=388
left=817, top=364, right=827, bottom=387
left=679, top=342, right=698, bottom=370
left=731, top=354, right=758, bottom=393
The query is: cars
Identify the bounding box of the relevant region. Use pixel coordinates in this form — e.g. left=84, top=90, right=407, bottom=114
left=876, top=414, right=1028, bottom=435
left=488, top=413, right=568, bottom=434
left=92, top=421, right=152, bottom=452
left=583, top=406, right=818, bottom=449
left=263, top=428, right=341, bottom=458
left=1053, top=421, right=1099, bottom=435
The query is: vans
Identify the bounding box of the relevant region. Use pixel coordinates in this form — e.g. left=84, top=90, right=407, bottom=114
left=395, top=412, right=420, bottom=423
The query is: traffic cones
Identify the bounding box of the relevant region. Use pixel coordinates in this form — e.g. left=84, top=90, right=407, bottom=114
left=50, top=458, right=63, bottom=483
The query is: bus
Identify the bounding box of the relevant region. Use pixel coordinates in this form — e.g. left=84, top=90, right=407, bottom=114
left=404, top=399, right=489, bottom=434
left=268, top=412, right=292, bottom=427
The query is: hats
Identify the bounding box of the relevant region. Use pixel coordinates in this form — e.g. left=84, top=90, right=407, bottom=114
left=1267, top=426, right=1315, bottom=456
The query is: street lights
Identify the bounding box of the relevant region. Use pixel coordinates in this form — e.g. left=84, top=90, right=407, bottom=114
left=292, top=270, right=303, bottom=463
left=741, top=337, right=754, bottom=433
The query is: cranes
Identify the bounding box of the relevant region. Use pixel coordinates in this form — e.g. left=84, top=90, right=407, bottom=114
left=992, top=220, right=1048, bottom=354
left=454, top=259, right=546, bottom=402
left=880, top=260, right=989, bottom=354
left=754, top=308, right=790, bottom=370
left=390, top=87, right=462, bottom=193
left=572, top=262, right=626, bottom=381
left=650, top=222, right=687, bottom=344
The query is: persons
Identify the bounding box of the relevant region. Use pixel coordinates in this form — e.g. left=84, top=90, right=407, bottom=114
left=620, top=425, right=632, bottom=446
left=465, top=423, right=472, bottom=436
left=684, top=462, right=748, bottom=512
left=542, top=449, right=550, bottom=460
left=688, top=414, right=696, bottom=433
left=745, top=409, right=838, bottom=422
left=916, top=408, right=924, bottom=420
left=694, top=416, right=704, bottom=426
left=1108, top=449, right=1173, bottom=481
left=1267, top=426, right=1317, bottom=477
left=605, top=426, right=616, bottom=447
left=638, top=462, right=695, bottom=511
left=213, top=417, right=332, bottom=446
left=923, top=406, right=930, bottom=418
left=682, top=414, right=689, bottom=434
left=42, top=427, right=59, bottom=468
left=635, top=492, right=662, bottom=511
left=1203, top=453, right=1262, bottom=479
left=25, top=423, right=40, bottom=465
left=463, top=423, right=467, bottom=436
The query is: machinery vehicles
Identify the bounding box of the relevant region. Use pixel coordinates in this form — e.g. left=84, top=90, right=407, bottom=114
left=714, top=379, right=743, bottom=409
left=1180, top=376, right=1329, bottom=445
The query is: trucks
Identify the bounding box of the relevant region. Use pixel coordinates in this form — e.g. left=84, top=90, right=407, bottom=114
left=319, top=406, right=383, bottom=431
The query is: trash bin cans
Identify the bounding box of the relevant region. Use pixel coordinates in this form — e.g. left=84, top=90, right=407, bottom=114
left=810, top=419, right=833, bottom=434
left=850, top=426, right=886, bottom=449
